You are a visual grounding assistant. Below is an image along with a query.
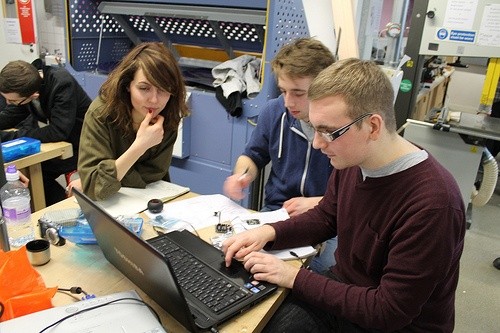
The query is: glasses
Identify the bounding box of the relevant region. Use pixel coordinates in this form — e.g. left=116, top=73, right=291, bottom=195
left=300, top=113, right=374, bottom=142
left=0, top=90, right=38, bottom=107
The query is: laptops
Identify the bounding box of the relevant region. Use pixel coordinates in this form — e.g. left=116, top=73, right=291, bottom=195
left=70, top=186, right=277, bottom=333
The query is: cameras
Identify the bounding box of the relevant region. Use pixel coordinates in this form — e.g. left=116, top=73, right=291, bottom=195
left=37, top=218, right=67, bottom=245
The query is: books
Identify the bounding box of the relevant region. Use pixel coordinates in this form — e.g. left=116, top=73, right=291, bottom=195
left=80, top=179, right=191, bottom=219
left=229, top=204, right=318, bottom=265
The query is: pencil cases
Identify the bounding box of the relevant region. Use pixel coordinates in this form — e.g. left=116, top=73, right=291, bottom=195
left=1, top=137, right=41, bottom=162
left=59, top=217, right=143, bottom=244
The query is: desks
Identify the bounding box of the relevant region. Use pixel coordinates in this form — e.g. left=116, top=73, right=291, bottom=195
left=444, top=111, right=500, bottom=142
left=0, top=138, right=326, bottom=333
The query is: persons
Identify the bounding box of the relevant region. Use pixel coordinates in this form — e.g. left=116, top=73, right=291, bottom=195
left=75, top=38, right=181, bottom=203
left=0, top=58, right=93, bottom=212
left=217, top=35, right=349, bottom=282
left=218, top=57, right=464, bottom=333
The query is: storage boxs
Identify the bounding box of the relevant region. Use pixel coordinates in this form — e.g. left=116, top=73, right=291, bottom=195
left=1, top=138, right=41, bottom=164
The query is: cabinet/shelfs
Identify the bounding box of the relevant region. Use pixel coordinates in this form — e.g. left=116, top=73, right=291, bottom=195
left=65, top=0, right=311, bottom=210
left=412, top=76, right=449, bottom=120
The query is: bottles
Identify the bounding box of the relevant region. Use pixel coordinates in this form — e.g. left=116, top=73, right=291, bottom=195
left=0, top=164, right=37, bottom=247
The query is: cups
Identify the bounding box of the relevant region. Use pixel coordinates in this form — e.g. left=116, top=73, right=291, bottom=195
left=26, top=239, right=51, bottom=266
